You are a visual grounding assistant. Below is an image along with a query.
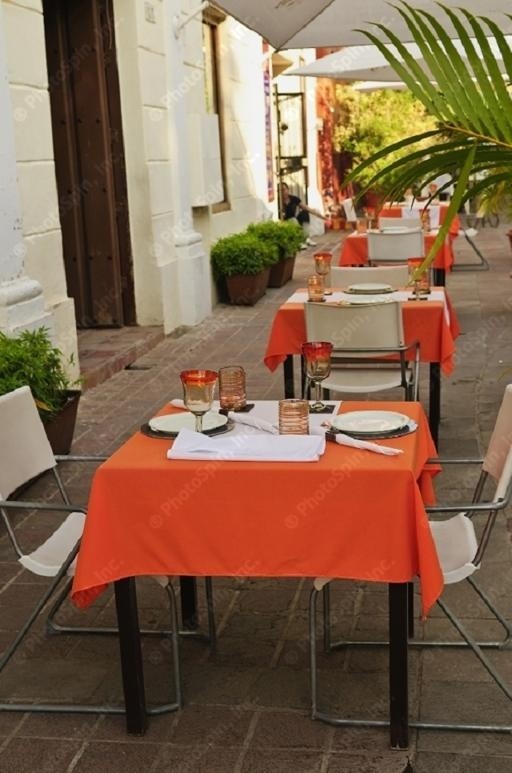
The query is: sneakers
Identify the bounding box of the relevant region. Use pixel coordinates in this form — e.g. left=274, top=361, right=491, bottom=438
left=301, top=238, right=317, bottom=249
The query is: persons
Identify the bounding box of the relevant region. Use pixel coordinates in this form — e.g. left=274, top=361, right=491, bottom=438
left=423, top=185, right=446, bottom=202
left=277, top=182, right=330, bottom=250
left=398, top=185, right=418, bottom=201
left=432, top=173, right=455, bottom=204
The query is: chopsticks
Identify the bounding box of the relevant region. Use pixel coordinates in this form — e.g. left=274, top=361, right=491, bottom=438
left=171, top=399, right=228, bottom=416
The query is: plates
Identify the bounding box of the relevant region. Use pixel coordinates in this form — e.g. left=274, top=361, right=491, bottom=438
left=349, top=283, right=391, bottom=291
left=334, top=411, right=409, bottom=434
left=148, top=413, right=228, bottom=435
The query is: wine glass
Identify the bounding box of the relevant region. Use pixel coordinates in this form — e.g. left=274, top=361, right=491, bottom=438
left=180, top=369, right=221, bottom=432
left=406, top=257, right=431, bottom=301
left=302, top=342, right=333, bottom=411
left=357, top=208, right=378, bottom=234
left=306, top=252, right=333, bottom=301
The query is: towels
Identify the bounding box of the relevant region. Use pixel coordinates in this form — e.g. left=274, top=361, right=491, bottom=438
left=167, top=427, right=325, bottom=463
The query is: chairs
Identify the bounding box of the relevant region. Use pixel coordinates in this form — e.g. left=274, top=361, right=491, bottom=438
left=303, top=299, right=420, bottom=402
left=330, top=217, right=425, bottom=286
left=0, top=386, right=215, bottom=713
left=310, top=383, right=512, bottom=734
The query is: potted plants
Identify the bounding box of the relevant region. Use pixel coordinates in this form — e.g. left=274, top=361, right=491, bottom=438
left=0, top=326, right=78, bottom=455
left=212, top=219, right=309, bottom=307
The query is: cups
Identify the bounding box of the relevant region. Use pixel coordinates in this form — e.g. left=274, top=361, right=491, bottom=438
left=279, top=399, right=309, bottom=435
left=218, top=364, right=246, bottom=411
left=418, top=208, right=431, bottom=233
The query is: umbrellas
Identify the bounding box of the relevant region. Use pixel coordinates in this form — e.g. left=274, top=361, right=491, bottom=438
left=204, top=0, right=512, bottom=42
left=283, top=43, right=511, bottom=82
left=353, top=82, right=442, bottom=93
